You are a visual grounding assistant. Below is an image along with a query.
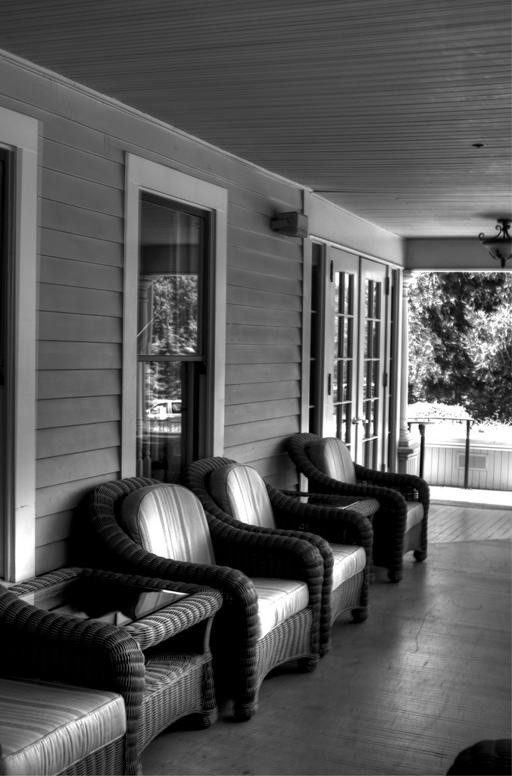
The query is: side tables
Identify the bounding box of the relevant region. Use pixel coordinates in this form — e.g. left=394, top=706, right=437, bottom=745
left=280, top=486, right=381, bottom=585
left=8, top=566, right=227, bottom=755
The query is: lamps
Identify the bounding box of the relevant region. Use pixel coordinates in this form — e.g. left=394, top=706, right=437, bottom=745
left=270, top=211, right=309, bottom=239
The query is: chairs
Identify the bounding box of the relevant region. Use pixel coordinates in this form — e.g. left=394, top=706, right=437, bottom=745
left=91, top=476, right=325, bottom=721
left=287, top=433, right=430, bottom=583
left=185, top=456, right=375, bottom=657
left=0, top=580, right=145, bottom=776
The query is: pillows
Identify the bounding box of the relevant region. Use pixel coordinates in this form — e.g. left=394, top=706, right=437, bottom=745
left=308, top=437, right=357, bottom=486
left=121, top=483, right=217, bottom=566
left=210, top=463, right=277, bottom=530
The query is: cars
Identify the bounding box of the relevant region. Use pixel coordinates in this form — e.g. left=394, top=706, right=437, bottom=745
left=146, top=399, right=183, bottom=422
